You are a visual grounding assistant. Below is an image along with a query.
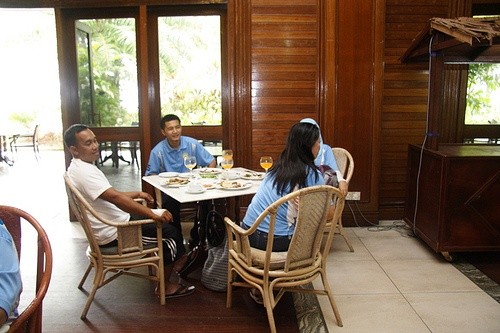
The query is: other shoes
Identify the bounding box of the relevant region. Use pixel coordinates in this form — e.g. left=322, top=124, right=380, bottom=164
left=250, top=290, right=264, bottom=304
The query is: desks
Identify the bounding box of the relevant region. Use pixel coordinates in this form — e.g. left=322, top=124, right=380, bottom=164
left=142, top=167, right=267, bottom=277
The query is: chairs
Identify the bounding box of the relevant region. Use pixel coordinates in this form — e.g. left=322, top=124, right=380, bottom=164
left=9, top=124, right=40, bottom=160
left=224, top=185, right=345, bottom=333
left=0, top=206, right=52, bottom=333
left=64, top=173, right=166, bottom=319
left=325, top=147, right=354, bottom=252
left=118, top=122, right=140, bottom=164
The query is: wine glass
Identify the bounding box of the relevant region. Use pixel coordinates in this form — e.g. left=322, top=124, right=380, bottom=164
left=260, top=155, right=273, bottom=172
left=184, top=156, right=197, bottom=173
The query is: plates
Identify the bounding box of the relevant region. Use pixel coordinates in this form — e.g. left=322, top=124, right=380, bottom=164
left=158, top=168, right=267, bottom=193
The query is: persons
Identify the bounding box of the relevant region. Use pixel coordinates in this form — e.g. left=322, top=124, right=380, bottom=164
left=144, top=112, right=217, bottom=256
left=294, top=117, right=348, bottom=223
left=0, top=219, right=22, bottom=333
left=244, top=120, right=337, bottom=272
left=65, top=123, right=199, bottom=298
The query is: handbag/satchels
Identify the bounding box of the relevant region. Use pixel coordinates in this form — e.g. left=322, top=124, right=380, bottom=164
left=201, top=232, right=231, bottom=292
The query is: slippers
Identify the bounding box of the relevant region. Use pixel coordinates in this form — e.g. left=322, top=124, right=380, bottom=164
left=158, top=284, right=195, bottom=298
left=177, top=246, right=200, bottom=275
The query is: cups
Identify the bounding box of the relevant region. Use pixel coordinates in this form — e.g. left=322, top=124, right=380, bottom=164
left=221, top=150, right=233, bottom=171
